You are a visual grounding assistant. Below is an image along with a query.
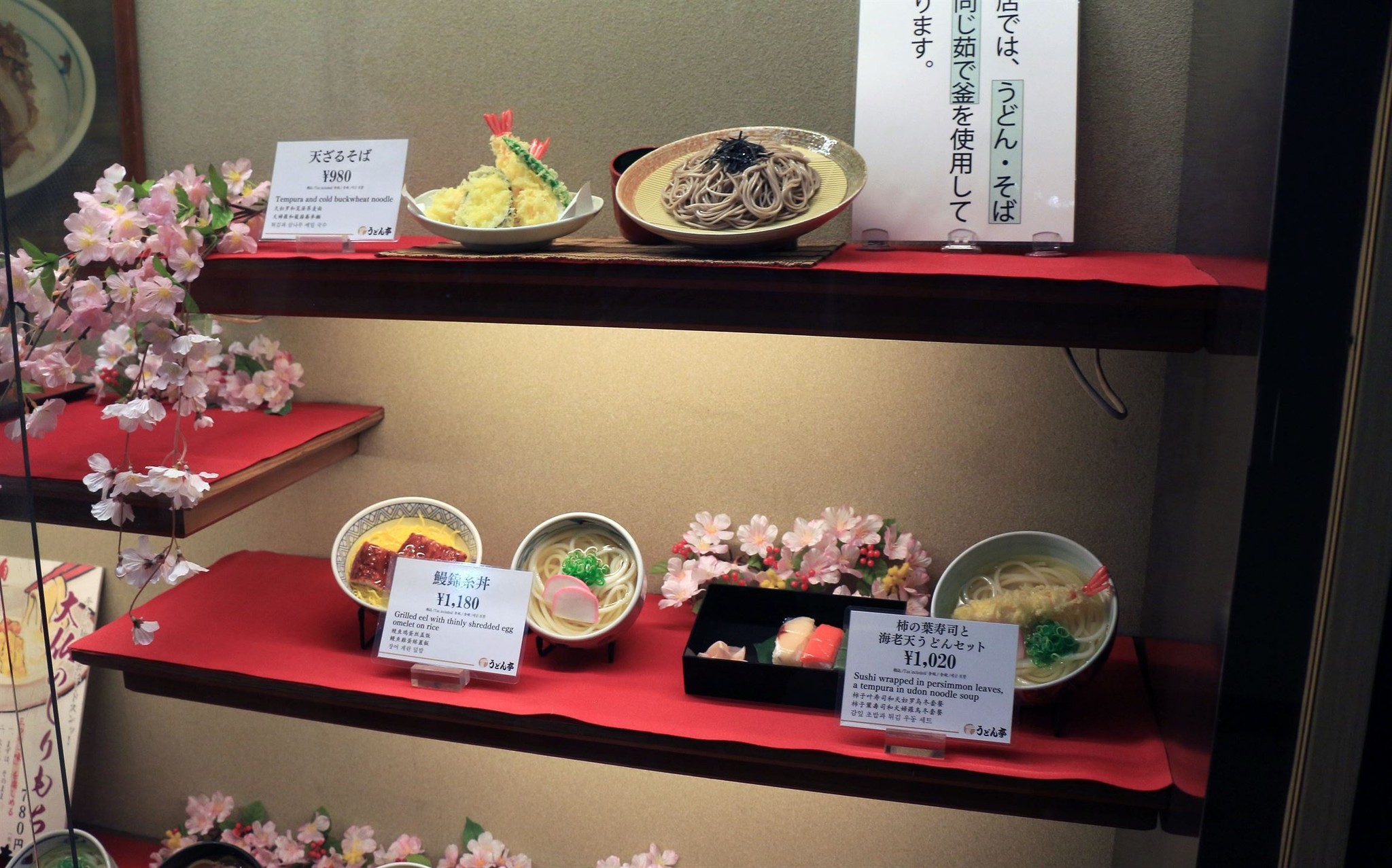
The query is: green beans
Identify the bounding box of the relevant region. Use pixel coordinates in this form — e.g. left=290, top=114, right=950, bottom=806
left=501, top=136, right=574, bottom=208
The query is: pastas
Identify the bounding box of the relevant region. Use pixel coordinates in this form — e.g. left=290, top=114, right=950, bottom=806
left=346, top=511, right=468, bottom=607
left=660, top=141, right=822, bottom=229
left=958, top=561, right=1109, bottom=685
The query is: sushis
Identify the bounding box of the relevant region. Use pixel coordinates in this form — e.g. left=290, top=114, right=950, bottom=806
left=696, top=616, right=850, bottom=671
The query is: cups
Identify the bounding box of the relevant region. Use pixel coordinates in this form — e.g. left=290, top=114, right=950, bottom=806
left=609, top=146, right=663, bottom=242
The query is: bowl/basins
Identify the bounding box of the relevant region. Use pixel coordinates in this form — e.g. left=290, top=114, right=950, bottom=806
left=509, top=513, right=647, bottom=650
left=330, top=496, right=483, bottom=612
left=931, top=530, right=1118, bottom=696
left=681, top=584, right=907, bottom=711
left=5, top=829, right=118, bottom=868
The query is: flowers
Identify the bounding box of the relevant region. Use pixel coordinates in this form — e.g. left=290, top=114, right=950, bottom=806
left=0, top=157, right=932, bottom=868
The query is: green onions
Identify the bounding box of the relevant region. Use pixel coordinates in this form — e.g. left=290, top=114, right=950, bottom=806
left=1023, top=619, right=1080, bottom=668
left=562, top=549, right=610, bottom=586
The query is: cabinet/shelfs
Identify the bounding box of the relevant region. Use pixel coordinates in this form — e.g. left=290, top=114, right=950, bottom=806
left=0, top=218, right=1266, bottom=834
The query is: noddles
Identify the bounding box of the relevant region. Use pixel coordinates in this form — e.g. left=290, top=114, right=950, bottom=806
left=527, top=529, right=636, bottom=636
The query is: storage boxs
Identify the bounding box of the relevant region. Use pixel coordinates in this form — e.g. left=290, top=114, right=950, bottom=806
left=682, top=584, right=907, bottom=713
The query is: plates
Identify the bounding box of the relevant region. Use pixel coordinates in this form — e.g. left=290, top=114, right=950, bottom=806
left=407, top=189, right=604, bottom=251
left=157, top=842, right=263, bottom=868
left=615, top=126, right=868, bottom=243
